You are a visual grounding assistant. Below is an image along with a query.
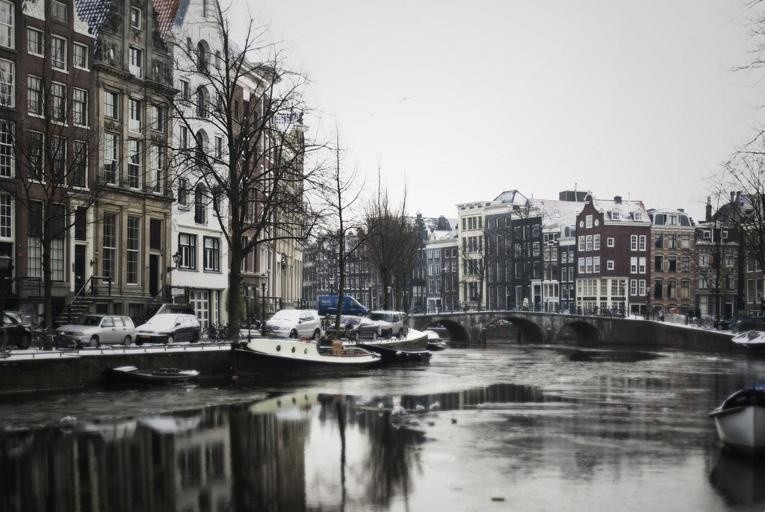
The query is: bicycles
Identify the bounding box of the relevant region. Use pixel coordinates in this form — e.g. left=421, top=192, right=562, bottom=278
left=207, top=320, right=242, bottom=342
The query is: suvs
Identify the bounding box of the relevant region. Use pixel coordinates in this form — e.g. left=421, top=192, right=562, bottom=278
left=398, top=311, right=409, bottom=337
left=367, top=311, right=404, bottom=339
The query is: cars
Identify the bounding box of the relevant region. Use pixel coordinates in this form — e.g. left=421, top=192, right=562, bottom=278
left=1, top=310, right=32, bottom=351
left=87, top=421, right=138, bottom=441
left=326, top=316, right=382, bottom=339
left=144, top=414, right=202, bottom=436
left=2, top=433, right=36, bottom=458
left=714, top=312, right=735, bottom=329
left=260, top=309, right=323, bottom=341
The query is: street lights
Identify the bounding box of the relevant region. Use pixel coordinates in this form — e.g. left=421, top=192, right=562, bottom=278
left=645, top=287, right=652, bottom=318
left=620, top=282, right=627, bottom=315
left=261, top=280, right=266, bottom=322
left=540, top=279, right=545, bottom=309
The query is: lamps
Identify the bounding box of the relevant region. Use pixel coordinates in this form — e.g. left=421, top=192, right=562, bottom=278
left=168, top=253, right=182, bottom=273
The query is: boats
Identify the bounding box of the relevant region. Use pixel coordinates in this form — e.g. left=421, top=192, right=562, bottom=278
left=234, top=338, right=382, bottom=378
left=247, top=392, right=322, bottom=422
left=115, top=383, right=198, bottom=393
left=708, top=446, right=764, bottom=512
left=356, top=343, right=432, bottom=367
left=107, top=365, right=200, bottom=383
left=710, top=387, right=765, bottom=449
left=423, top=330, right=446, bottom=349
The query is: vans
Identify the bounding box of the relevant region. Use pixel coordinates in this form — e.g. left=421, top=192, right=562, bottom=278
left=315, top=295, right=369, bottom=317
left=134, top=313, right=201, bottom=344
left=57, top=314, right=136, bottom=348
